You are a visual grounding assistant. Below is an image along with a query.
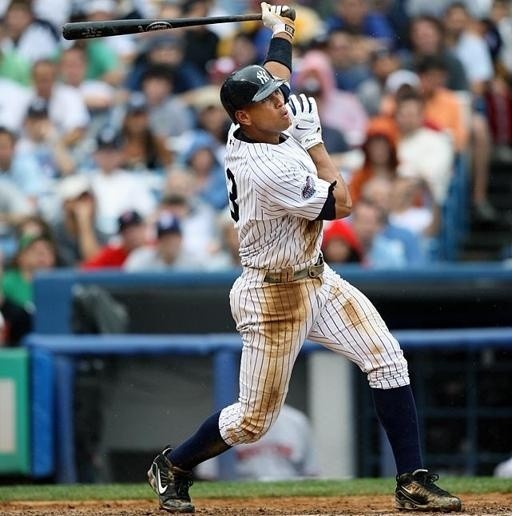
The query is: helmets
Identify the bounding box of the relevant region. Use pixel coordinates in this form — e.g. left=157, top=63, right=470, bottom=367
left=220, top=64, right=288, bottom=125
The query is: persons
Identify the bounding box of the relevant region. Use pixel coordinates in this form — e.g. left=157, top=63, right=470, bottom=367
left=145, top=2, right=463, bottom=514
left=0, top=1, right=512, bottom=346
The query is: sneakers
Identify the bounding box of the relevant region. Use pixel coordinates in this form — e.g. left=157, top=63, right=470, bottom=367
left=394, top=470, right=460, bottom=511
left=147, top=446, right=195, bottom=513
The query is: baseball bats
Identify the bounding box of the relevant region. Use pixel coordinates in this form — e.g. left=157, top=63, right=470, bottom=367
left=63, top=10, right=296, bottom=40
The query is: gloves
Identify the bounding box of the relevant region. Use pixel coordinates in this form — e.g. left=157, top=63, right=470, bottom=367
left=281, top=93, right=324, bottom=153
left=260, top=2, right=296, bottom=39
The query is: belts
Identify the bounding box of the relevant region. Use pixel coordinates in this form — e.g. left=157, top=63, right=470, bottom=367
left=264, top=256, right=324, bottom=283
